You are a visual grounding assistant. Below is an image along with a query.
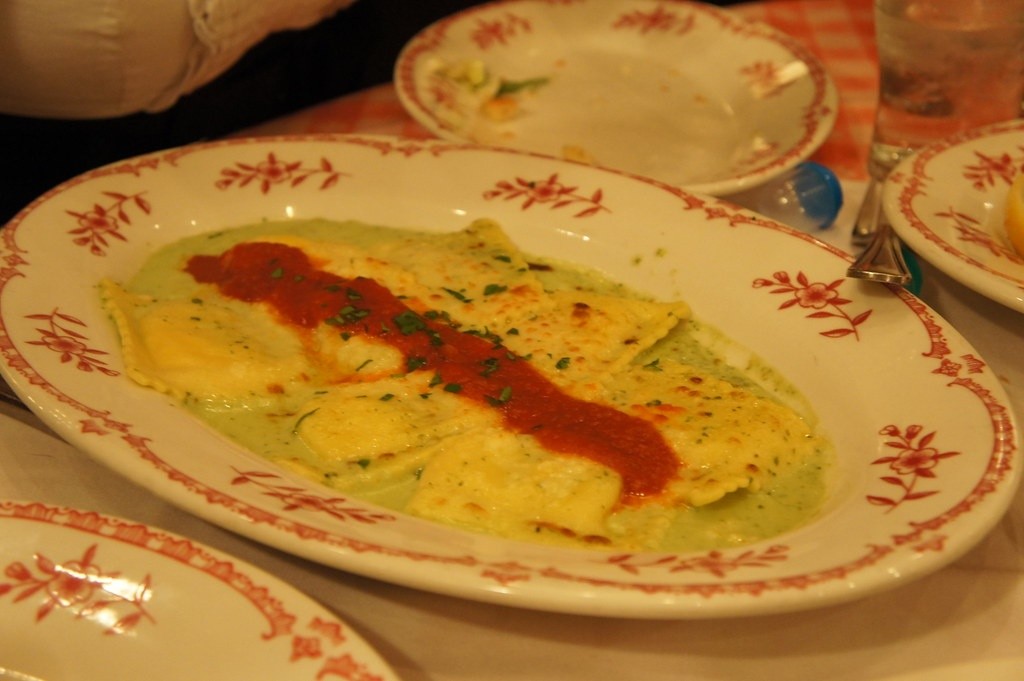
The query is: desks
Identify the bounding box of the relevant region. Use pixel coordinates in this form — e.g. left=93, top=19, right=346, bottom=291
left=0, top=0, right=1024, bottom=681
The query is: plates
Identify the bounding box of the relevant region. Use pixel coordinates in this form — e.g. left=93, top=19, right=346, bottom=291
left=392, top=1, right=838, bottom=196
left=0, top=133, right=1020, bottom=618
left=881, top=118, right=1024, bottom=311
left=0, top=498, right=402, bottom=681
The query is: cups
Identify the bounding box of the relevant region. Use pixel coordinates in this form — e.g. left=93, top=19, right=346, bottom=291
left=867, top=0, right=1024, bottom=184
